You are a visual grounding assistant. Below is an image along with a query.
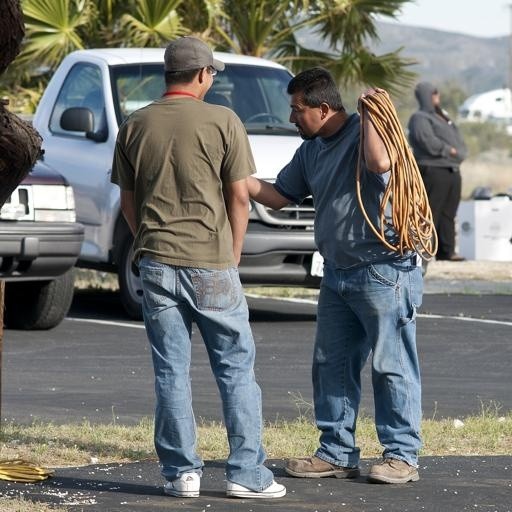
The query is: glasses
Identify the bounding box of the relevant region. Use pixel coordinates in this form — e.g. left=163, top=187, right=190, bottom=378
left=208, top=67, right=218, bottom=76
left=432, top=90, right=438, bottom=95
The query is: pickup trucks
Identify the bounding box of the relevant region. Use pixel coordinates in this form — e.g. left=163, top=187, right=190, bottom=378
left=31, top=47, right=325, bottom=320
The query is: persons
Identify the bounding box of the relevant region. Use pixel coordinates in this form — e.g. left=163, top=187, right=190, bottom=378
left=112, top=36, right=287, bottom=498
left=408, top=80, right=466, bottom=261
left=247, top=66, right=440, bottom=485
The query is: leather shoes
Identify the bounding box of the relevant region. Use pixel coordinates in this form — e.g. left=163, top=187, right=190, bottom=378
left=451, top=255, right=464, bottom=260
left=285, top=457, right=360, bottom=479
left=368, top=460, right=419, bottom=485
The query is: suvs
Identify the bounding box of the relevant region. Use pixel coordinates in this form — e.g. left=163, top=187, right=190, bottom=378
left=0, top=160, right=86, bottom=330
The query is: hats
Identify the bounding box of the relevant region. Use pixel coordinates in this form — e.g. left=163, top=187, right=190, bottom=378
left=164, top=37, right=225, bottom=72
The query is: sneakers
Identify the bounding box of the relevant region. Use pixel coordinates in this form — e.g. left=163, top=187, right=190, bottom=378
left=164, top=473, right=201, bottom=498
left=227, top=480, right=287, bottom=498
